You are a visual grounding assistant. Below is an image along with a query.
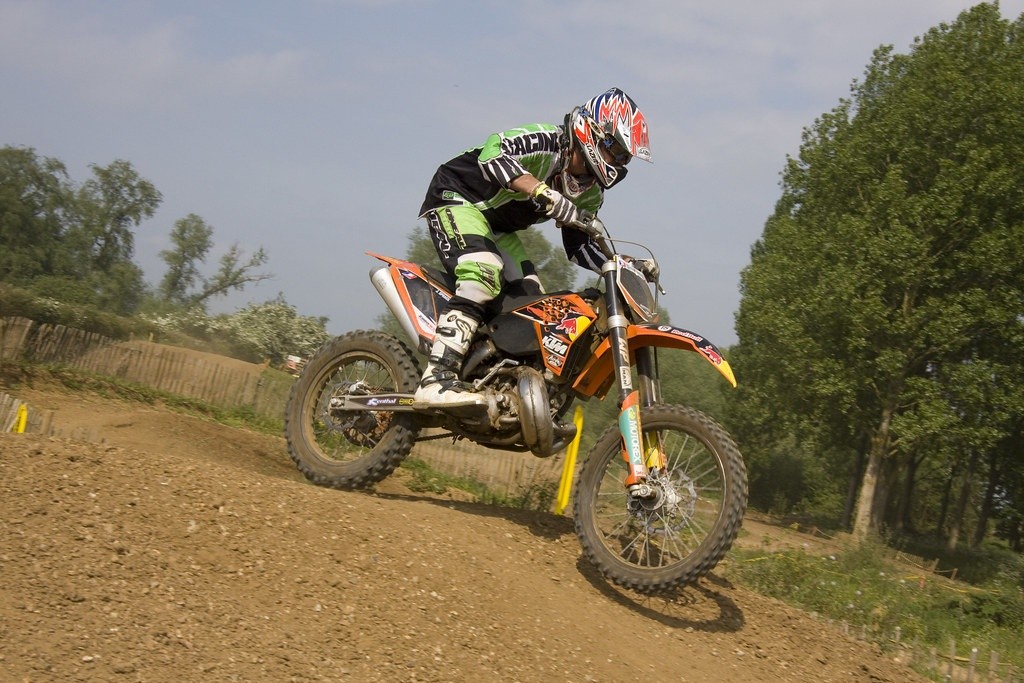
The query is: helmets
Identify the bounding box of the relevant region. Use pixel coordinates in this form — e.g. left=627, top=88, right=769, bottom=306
left=571, top=87, right=654, bottom=190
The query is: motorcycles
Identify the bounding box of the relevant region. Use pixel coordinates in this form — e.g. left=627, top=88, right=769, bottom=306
left=284, top=202, right=750, bottom=595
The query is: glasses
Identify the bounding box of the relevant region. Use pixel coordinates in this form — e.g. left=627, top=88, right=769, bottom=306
left=602, top=134, right=632, bottom=165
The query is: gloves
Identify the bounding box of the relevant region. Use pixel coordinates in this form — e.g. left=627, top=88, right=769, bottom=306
left=635, top=260, right=660, bottom=282
left=530, top=182, right=577, bottom=228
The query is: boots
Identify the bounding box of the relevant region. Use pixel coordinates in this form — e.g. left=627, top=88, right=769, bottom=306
left=413, top=302, right=489, bottom=415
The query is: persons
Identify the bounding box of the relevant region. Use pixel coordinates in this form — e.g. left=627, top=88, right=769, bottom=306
left=412, top=88, right=660, bottom=416
